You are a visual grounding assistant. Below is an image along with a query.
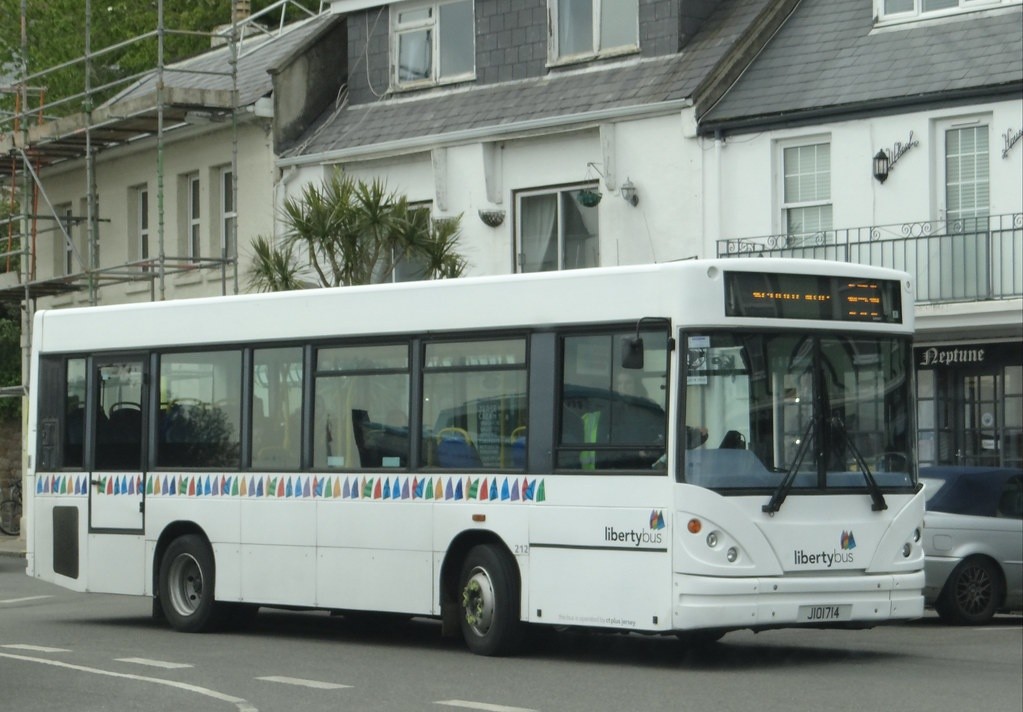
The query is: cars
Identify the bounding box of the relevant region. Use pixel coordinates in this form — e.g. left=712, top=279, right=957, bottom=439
left=917, top=466, right=1023, bottom=625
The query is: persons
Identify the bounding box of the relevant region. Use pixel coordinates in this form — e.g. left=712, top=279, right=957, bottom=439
left=594, top=370, right=708, bottom=469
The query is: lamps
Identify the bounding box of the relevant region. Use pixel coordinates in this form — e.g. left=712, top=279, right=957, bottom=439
left=621, top=177, right=639, bottom=207
left=872, top=130, right=918, bottom=185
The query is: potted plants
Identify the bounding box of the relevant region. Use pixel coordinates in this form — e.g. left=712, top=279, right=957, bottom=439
left=576, top=189, right=603, bottom=209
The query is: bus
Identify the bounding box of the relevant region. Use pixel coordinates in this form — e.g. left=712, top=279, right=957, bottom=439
left=23, top=257, right=928, bottom=655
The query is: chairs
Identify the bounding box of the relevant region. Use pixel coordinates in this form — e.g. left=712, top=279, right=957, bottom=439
left=510, top=425, right=529, bottom=470
left=66, top=398, right=144, bottom=464
left=433, top=428, right=476, bottom=470
